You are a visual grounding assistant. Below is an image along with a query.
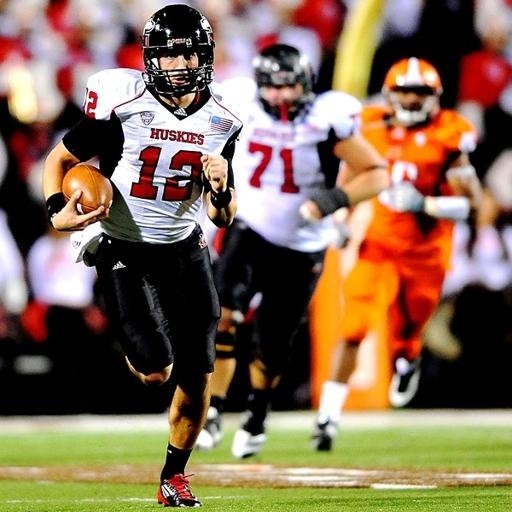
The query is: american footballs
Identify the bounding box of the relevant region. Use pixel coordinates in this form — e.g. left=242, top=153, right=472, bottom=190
left=62, top=164, right=113, bottom=216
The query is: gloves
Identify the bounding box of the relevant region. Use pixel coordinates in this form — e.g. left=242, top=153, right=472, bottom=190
left=377, top=181, right=423, bottom=214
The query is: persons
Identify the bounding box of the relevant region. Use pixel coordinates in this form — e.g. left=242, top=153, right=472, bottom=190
left=43, top=4, right=243, bottom=507
left=195, top=44, right=389, bottom=457
left=0, top=0, right=511, bottom=360
left=310, top=59, right=483, bottom=453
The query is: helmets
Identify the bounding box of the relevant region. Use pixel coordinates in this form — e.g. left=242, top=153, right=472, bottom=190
left=249, top=43, right=318, bottom=120
left=140, top=3, right=217, bottom=94
left=380, top=54, right=444, bottom=126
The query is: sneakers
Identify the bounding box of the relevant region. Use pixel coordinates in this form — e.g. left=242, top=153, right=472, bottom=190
left=154, top=472, right=202, bottom=510
left=232, top=428, right=268, bottom=458
left=194, top=405, right=226, bottom=449
left=387, top=357, right=421, bottom=409
left=310, top=413, right=338, bottom=455
